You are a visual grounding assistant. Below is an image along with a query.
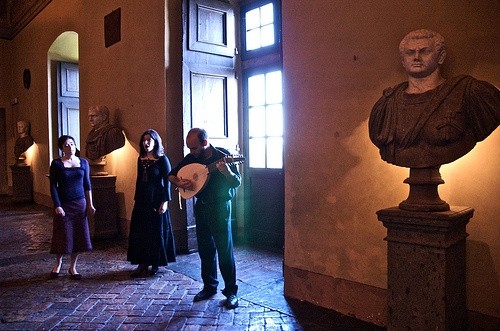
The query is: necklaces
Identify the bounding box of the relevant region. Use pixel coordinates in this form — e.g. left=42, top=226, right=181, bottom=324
left=146, top=155, right=153, bottom=160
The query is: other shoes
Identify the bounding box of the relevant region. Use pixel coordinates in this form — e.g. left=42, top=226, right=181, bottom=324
left=227, top=294, right=238, bottom=307
left=194, top=290, right=212, bottom=300
left=69, top=269, right=81, bottom=279
left=51, top=264, right=63, bottom=277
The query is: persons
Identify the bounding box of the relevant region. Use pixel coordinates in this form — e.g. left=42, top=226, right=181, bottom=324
left=86, top=105, right=125, bottom=160
left=368, top=29, right=500, bottom=167
left=167, top=128, right=241, bottom=308
left=50, top=135, right=96, bottom=280
left=126, top=129, right=176, bottom=277
left=14, top=120, right=36, bottom=158
